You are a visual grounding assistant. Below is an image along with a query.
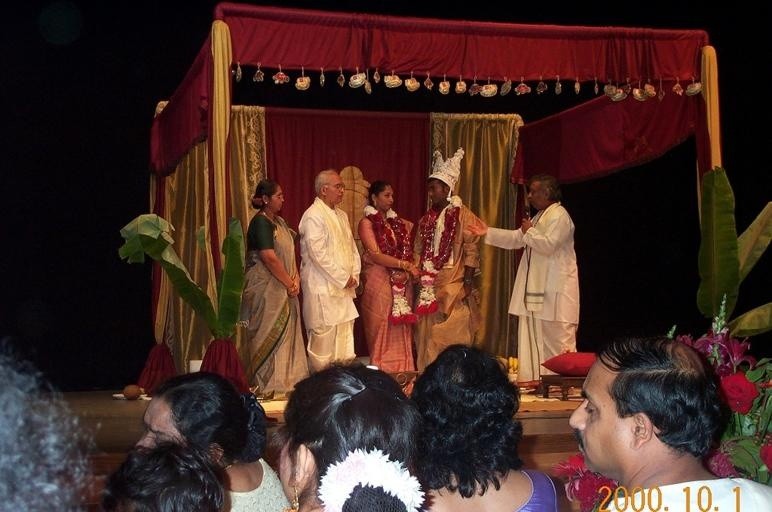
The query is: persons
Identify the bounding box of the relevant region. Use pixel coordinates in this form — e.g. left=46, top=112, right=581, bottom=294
left=408, top=343, right=568, bottom=511
left=135, top=371, right=292, bottom=512
left=466, top=174, right=580, bottom=394
left=357, top=178, right=419, bottom=398
left=102, top=434, right=225, bottom=512
left=565, top=334, right=772, bottom=512
left=410, top=148, right=482, bottom=378
left=274, top=359, right=426, bottom=512
left=238, top=179, right=310, bottom=402
left=298, top=169, right=361, bottom=373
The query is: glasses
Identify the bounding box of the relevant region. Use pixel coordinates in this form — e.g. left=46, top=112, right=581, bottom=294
left=323, top=183, right=345, bottom=189
left=272, top=194, right=284, bottom=199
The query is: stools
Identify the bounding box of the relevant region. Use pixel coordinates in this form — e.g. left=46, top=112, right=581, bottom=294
left=541, top=375, right=587, bottom=401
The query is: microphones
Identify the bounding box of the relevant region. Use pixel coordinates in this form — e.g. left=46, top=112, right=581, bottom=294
left=523, top=206, right=530, bottom=221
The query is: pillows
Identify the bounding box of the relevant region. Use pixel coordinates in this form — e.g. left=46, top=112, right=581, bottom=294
left=541, top=353, right=594, bottom=374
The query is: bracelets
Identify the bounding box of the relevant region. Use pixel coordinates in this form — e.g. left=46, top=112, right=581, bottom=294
left=399, top=260, right=408, bottom=271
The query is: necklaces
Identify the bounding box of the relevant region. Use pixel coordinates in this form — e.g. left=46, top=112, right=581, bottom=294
left=219, top=457, right=240, bottom=472
